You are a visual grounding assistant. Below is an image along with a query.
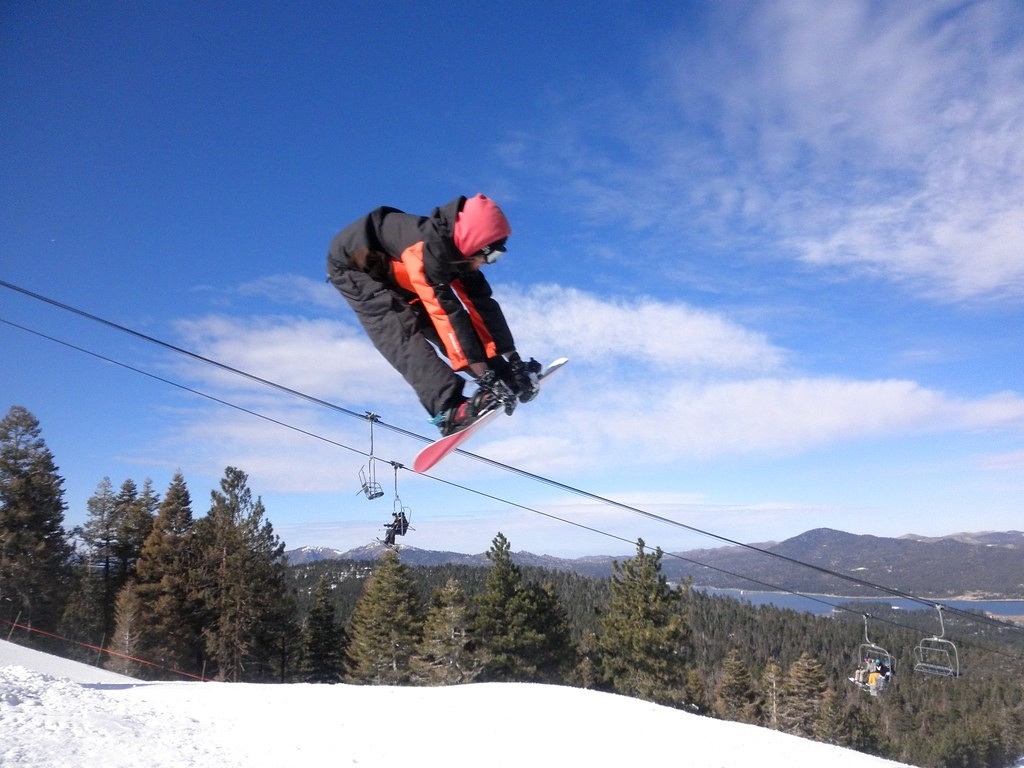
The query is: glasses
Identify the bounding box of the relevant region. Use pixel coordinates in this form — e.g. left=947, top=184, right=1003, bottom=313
left=481, top=245, right=507, bottom=264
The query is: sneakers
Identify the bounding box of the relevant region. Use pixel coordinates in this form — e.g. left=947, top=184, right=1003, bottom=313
left=436, top=387, right=499, bottom=437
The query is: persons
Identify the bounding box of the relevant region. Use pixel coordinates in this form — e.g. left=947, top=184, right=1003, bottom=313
left=855, top=656, right=893, bottom=690
left=327, top=193, right=540, bottom=435
left=383, top=512, right=409, bottom=548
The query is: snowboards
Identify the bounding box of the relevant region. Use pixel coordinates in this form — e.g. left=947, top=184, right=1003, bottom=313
left=409, top=356, right=570, bottom=475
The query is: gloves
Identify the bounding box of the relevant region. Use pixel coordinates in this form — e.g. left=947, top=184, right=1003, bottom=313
left=504, top=352, right=543, bottom=403
left=473, top=370, right=519, bottom=416
